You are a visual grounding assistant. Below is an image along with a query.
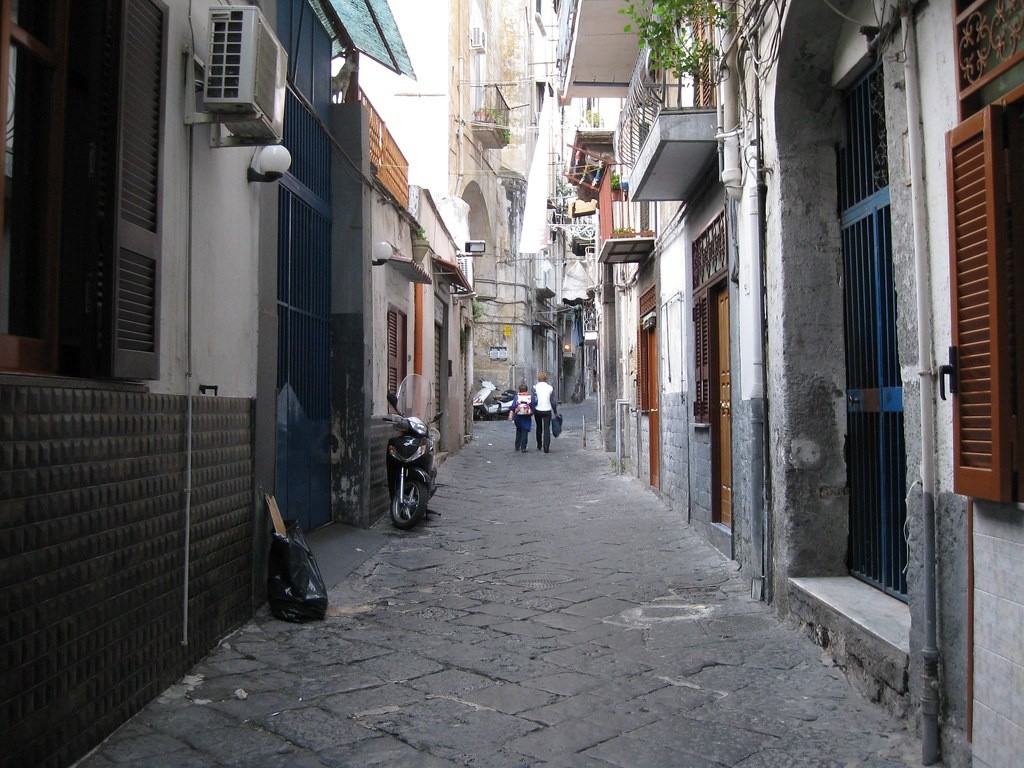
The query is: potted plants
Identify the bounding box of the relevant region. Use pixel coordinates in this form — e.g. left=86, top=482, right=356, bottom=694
left=610, top=173, right=621, bottom=202
left=472, top=106, right=497, bottom=123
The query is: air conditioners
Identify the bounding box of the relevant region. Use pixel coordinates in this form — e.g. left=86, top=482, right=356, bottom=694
left=457, top=258, right=475, bottom=292
left=202, top=4, right=289, bottom=140
left=471, top=27, right=486, bottom=54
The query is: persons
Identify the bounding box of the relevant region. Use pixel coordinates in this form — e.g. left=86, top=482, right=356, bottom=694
left=508, top=383, right=536, bottom=452
left=533, top=371, right=562, bottom=453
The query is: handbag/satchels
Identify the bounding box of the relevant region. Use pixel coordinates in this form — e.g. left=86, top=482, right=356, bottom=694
left=552, top=414, right=562, bottom=437
left=533, top=385, right=537, bottom=406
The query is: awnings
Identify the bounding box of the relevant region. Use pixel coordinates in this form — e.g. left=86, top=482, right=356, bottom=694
left=302, top=0, right=418, bottom=84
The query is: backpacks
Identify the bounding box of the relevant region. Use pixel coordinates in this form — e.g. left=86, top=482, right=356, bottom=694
left=514, top=391, right=533, bottom=416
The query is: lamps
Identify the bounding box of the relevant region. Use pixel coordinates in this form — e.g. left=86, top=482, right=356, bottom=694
left=372, top=241, right=394, bottom=266
left=247, top=144, right=291, bottom=184
left=465, top=239, right=486, bottom=253
left=564, top=344, right=570, bottom=352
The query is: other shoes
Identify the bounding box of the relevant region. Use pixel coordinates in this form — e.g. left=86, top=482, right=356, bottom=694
left=522, top=449, right=525, bottom=452
left=544, top=447, right=549, bottom=453
left=538, top=446, right=541, bottom=449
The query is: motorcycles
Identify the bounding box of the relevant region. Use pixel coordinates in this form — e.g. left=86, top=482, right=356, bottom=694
left=381, top=374, right=445, bottom=531
left=473, top=378, right=518, bottom=422
